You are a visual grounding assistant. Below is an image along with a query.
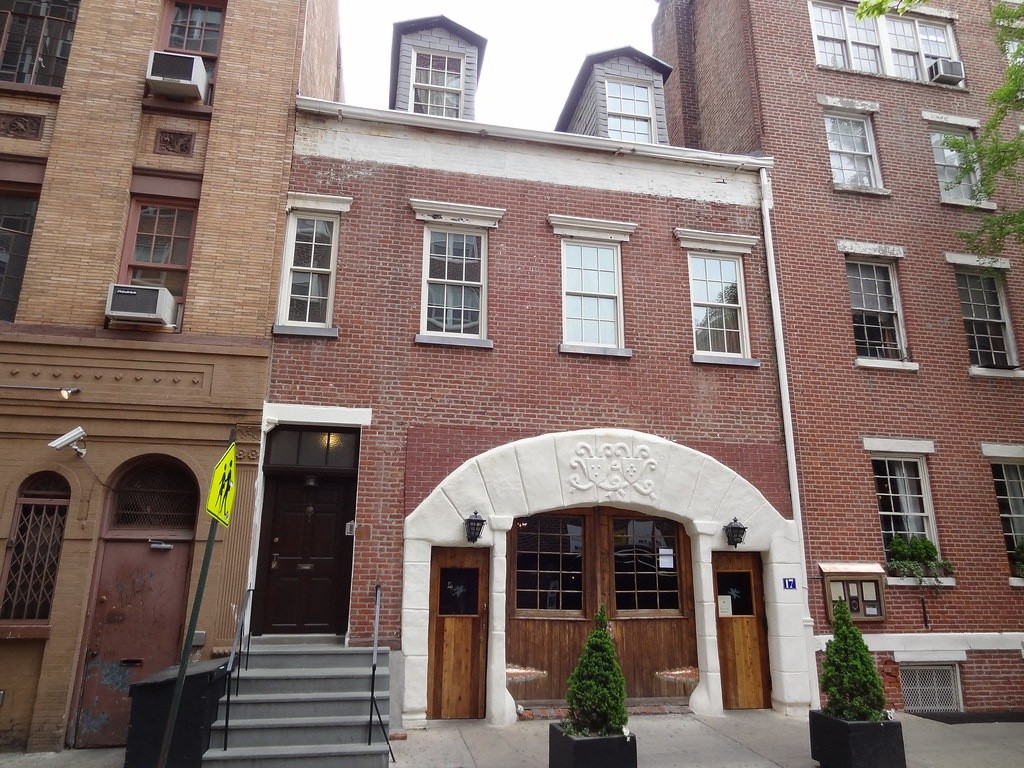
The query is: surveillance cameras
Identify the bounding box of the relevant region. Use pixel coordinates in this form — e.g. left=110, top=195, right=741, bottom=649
left=48, top=426, right=87, bottom=451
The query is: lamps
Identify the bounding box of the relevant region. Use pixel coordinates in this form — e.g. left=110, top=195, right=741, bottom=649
left=61, top=387, right=81, bottom=399
left=724, top=517, right=748, bottom=548
left=466, top=510, right=486, bottom=544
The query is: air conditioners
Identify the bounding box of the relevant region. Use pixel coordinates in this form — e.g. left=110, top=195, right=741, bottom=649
left=929, top=58, right=965, bottom=86
left=146, top=51, right=209, bottom=102
left=105, top=284, right=178, bottom=324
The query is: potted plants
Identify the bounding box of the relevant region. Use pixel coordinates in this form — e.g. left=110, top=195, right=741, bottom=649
left=808, top=595, right=907, bottom=768
left=887, top=532, right=953, bottom=585
left=549, top=603, right=636, bottom=768
